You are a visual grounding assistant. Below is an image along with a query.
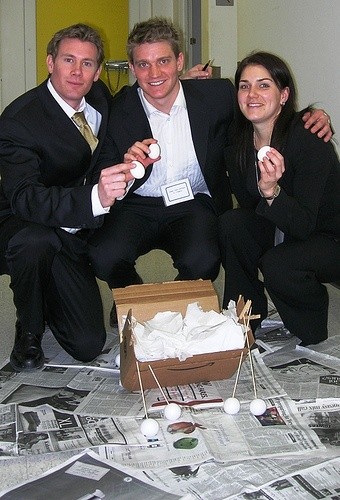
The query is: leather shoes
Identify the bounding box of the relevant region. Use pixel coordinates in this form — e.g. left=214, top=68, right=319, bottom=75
left=110, top=279, right=144, bottom=328
left=9, top=323, right=45, bottom=372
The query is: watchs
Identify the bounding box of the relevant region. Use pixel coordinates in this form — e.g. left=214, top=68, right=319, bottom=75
left=257, top=181, right=281, bottom=200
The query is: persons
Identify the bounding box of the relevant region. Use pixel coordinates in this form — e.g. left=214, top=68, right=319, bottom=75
left=84, top=18, right=336, bottom=327
left=0, top=24, right=215, bottom=373
left=215, top=51, right=339, bottom=348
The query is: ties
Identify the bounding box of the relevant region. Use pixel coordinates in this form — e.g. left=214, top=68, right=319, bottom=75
left=70, top=112, right=99, bottom=155
